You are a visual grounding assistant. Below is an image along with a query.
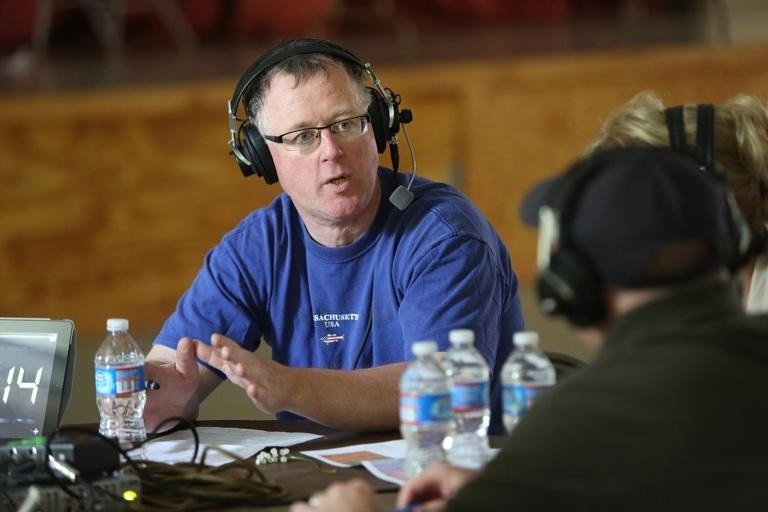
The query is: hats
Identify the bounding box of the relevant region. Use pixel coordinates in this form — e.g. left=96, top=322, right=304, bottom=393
left=520, top=146, right=734, bottom=286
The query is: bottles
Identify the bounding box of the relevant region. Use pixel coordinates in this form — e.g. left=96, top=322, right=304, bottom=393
left=396, top=340, right=455, bottom=481
left=91, top=317, right=150, bottom=444
left=441, top=327, right=496, bottom=469
left=500, top=330, right=556, bottom=438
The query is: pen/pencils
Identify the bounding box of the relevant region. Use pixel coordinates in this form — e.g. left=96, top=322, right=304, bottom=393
left=146, top=380, right=161, bottom=391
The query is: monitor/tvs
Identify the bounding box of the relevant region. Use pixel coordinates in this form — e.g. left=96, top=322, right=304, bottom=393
left=1, top=317, right=77, bottom=444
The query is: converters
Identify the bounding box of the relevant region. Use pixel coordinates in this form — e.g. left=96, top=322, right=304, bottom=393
left=2, top=434, right=122, bottom=484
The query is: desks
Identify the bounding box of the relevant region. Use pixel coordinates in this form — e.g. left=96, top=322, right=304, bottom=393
left=56, top=419, right=505, bottom=512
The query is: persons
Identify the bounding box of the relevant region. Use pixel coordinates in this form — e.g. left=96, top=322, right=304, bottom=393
left=285, top=91, right=767, bottom=511
left=129, top=41, right=524, bottom=432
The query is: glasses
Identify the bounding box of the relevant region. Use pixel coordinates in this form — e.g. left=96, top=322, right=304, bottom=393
left=263, top=113, right=373, bottom=150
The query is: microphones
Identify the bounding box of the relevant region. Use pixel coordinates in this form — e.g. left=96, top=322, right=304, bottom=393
left=388, top=109, right=417, bottom=210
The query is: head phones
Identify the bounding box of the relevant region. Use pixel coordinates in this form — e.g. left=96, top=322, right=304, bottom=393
left=533, top=142, right=754, bottom=327
left=226, top=37, right=400, bottom=186
